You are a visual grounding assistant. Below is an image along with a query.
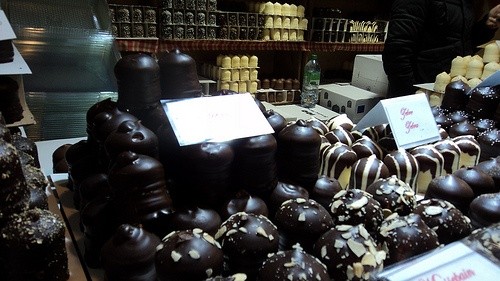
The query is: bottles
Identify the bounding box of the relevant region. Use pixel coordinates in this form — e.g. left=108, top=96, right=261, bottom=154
left=301, top=54, right=321, bottom=108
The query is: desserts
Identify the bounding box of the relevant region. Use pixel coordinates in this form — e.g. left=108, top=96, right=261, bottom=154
left=0, top=125, right=71, bottom=281
left=107, top=0, right=379, bottom=44
left=53, top=40, right=500, bottom=281
